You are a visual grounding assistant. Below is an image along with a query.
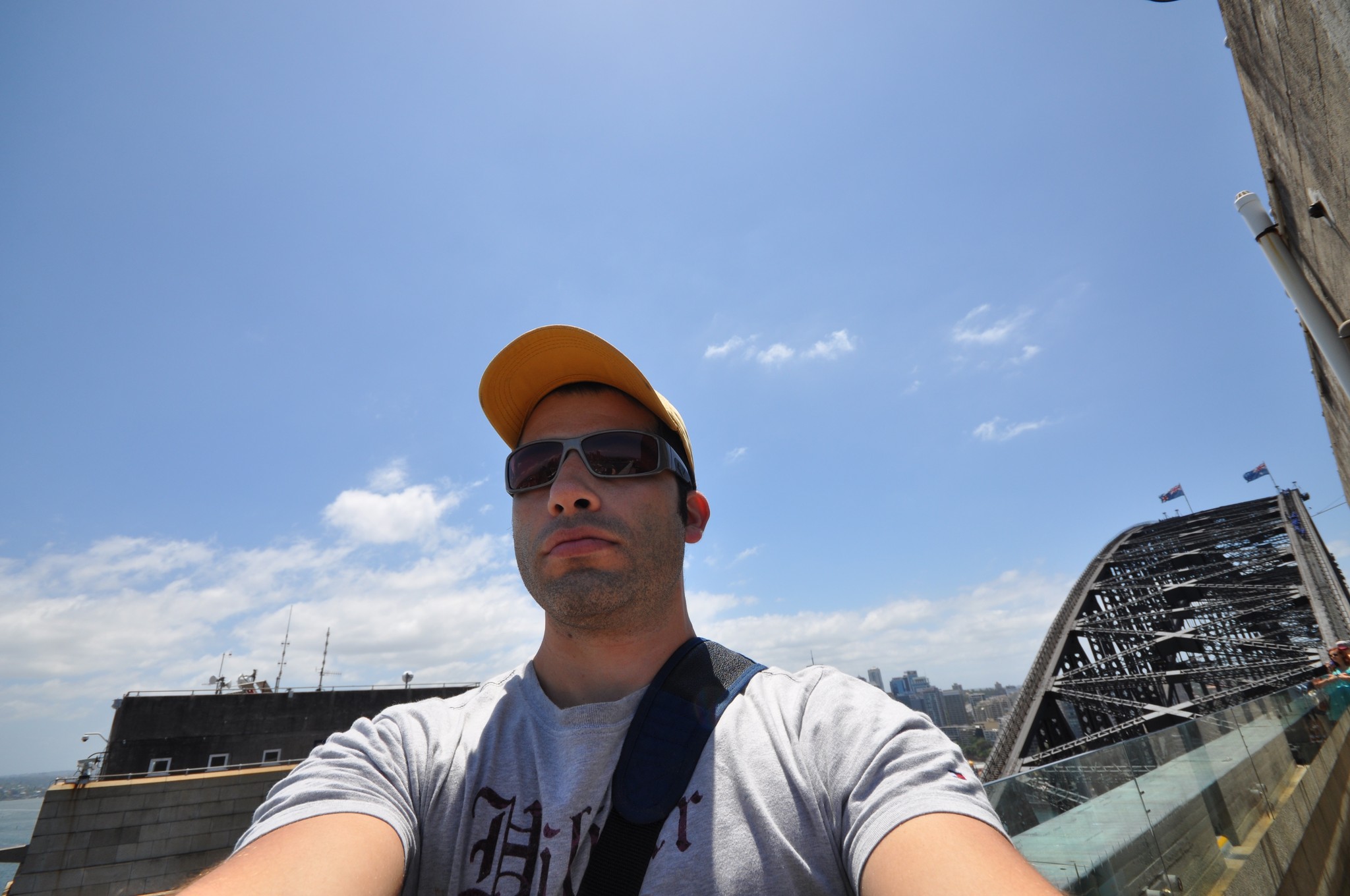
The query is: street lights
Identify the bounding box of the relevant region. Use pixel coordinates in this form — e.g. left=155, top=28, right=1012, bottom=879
left=214, top=654, right=232, bottom=695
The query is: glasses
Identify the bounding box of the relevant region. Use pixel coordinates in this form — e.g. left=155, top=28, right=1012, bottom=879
left=1328, top=653, right=1340, bottom=657
left=504, top=428, right=694, bottom=497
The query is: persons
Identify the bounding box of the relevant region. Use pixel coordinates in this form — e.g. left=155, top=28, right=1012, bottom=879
left=1311, top=639, right=1350, bottom=684
left=178, top=325, right=1065, bottom=896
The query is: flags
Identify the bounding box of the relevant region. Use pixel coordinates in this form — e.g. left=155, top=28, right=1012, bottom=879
left=1243, top=463, right=1270, bottom=482
left=1158, top=485, right=1185, bottom=503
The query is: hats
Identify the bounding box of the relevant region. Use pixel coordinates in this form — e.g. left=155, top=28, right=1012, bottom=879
left=478, top=324, right=695, bottom=473
left=1335, top=640, right=1350, bottom=649
